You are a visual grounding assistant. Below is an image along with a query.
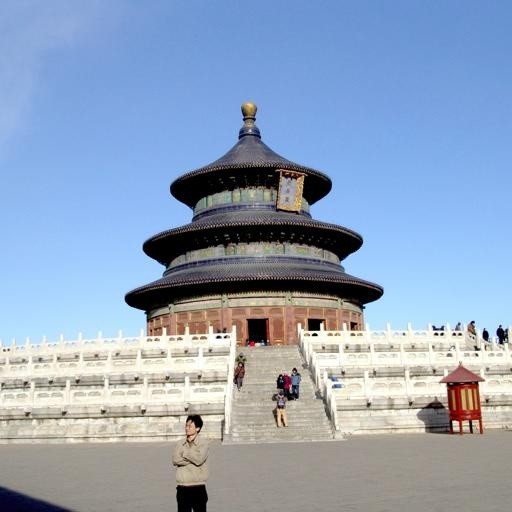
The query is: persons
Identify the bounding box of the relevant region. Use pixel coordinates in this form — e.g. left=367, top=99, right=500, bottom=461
left=290, top=368, right=302, bottom=400
left=245, top=339, right=255, bottom=347
left=452, top=320, right=509, bottom=351
left=223, top=327, right=227, bottom=338
left=282, top=372, right=292, bottom=400
left=236, top=352, right=247, bottom=367
left=276, top=392, right=288, bottom=428
left=277, top=374, right=286, bottom=397
left=173, top=414, right=211, bottom=512
left=235, top=362, right=245, bottom=391
left=215, top=327, right=221, bottom=338
